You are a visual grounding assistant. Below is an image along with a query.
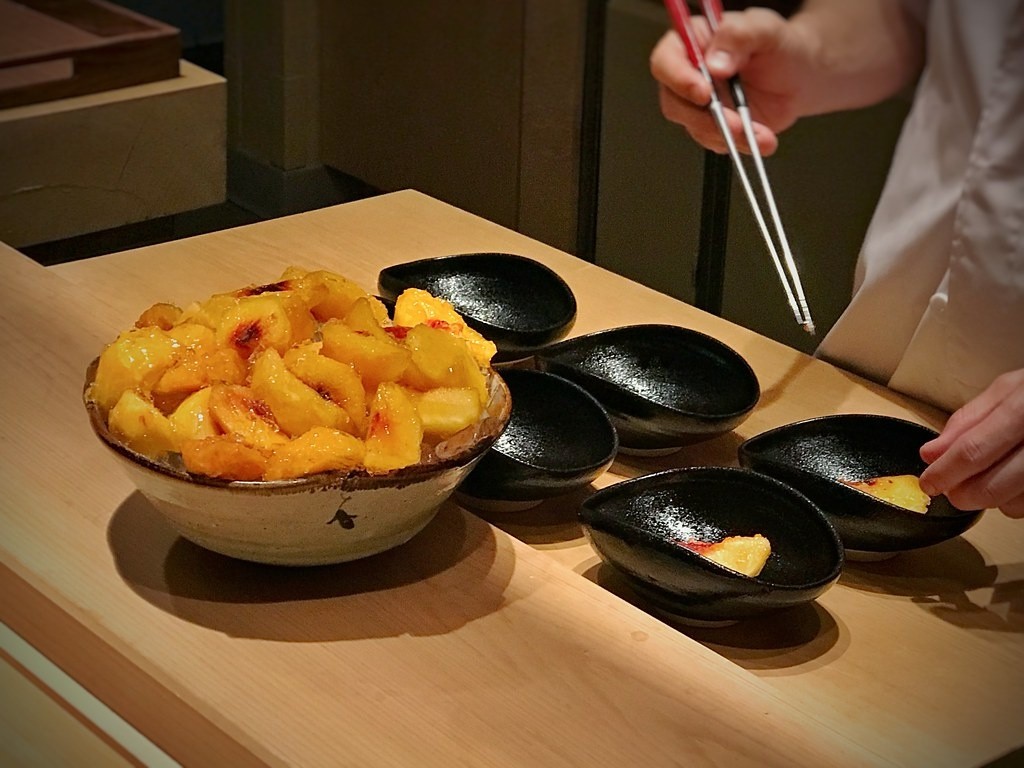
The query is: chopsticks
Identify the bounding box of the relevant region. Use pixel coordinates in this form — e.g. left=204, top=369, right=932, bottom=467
left=664, top=0, right=817, bottom=338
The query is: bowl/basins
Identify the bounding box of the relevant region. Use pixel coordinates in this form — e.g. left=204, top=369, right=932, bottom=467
left=452, top=365, right=618, bottom=512
left=535, top=323, right=762, bottom=456
left=577, top=466, right=846, bottom=624
left=733, top=413, right=988, bottom=563
left=377, top=252, right=578, bottom=356
left=84, top=355, right=514, bottom=566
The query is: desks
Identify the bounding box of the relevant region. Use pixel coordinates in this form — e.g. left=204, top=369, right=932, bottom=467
left=0, top=183, right=1024, bottom=768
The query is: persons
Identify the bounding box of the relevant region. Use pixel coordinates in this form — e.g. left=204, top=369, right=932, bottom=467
left=651, top=0, right=1024, bottom=520
